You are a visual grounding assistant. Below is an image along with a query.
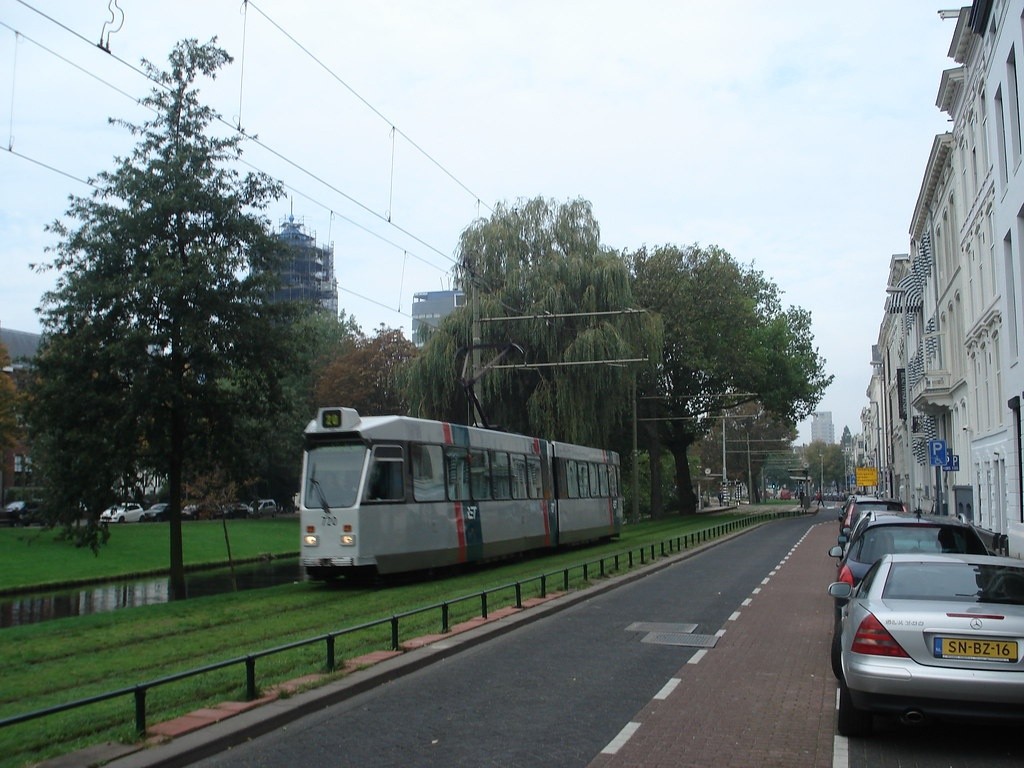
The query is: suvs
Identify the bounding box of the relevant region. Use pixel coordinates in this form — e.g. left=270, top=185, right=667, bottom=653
left=828, top=510, right=993, bottom=636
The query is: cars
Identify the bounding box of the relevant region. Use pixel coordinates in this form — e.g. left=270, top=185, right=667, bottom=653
left=221, top=501, right=248, bottom=520
left=828, top=554, right=1024, bottom=736
left=837, top=492, right=964, bottom=566
left=780, top=489, right=792, bottom=500
left=181, top=503, right=224, bottom=521
left=100, top=501, right=144, bottom=524
left=0, top=500, right=59, bottom=528
left=143, top=502, right=171, bottom=523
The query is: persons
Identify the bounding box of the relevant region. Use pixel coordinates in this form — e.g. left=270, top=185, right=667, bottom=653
left=799, top=489, right=825, bottom=508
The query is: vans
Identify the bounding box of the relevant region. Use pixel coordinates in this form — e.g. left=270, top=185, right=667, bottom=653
left=248, top=498, right=277, bottom=520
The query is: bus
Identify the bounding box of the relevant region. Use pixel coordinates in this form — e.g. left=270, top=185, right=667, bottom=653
left=297, top=340, right=627, bottom=592
